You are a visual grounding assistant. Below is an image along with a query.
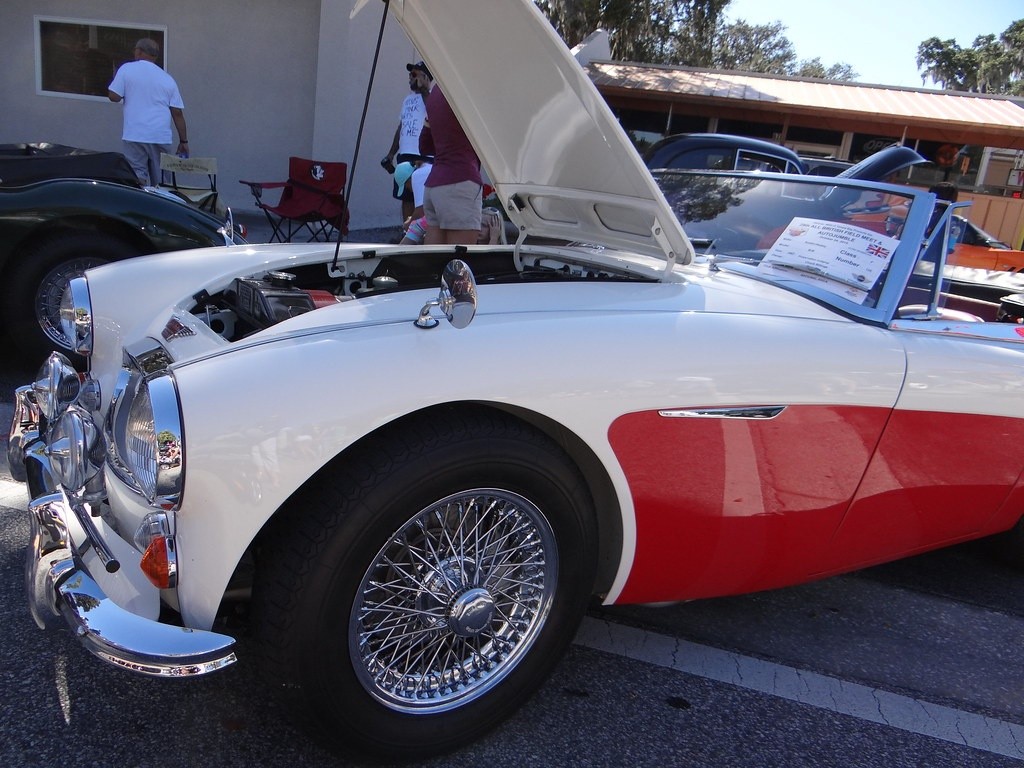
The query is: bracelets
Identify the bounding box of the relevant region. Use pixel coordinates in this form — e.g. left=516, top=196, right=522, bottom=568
left=180, top=140, right=188, bottom=144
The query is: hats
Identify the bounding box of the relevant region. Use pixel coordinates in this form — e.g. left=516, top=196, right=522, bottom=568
left=405, top=61, right=433, bottom=82
left=394, top=161, right=417, bottom=196
left=135, top=38, right=159, bottom=56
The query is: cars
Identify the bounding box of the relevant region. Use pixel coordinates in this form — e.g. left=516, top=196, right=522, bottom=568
left=641, top=132, right=1023, bottom=272
left=0, top=141, right=251, bottom=394
left=10, top=0, right=1023, bottom=756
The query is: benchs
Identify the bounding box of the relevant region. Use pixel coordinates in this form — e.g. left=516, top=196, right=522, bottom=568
left=900, top=287, right=1000, bottom=323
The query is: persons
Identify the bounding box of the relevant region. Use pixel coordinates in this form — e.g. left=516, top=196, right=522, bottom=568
left=891, top=181, right=961, bottom=240
left=108, top=39, right=190, bottom=188
left=381, top=61, right=507, bottom=245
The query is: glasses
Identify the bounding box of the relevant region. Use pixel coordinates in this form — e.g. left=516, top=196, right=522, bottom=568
left=482, top=207, right=498, bottom=213
left=409, top=71, right=425, bottom=78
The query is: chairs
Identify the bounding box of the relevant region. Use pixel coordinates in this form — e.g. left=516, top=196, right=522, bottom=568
left=896, top=303, right=985, bottom=323
left=157, top=152, right=226, bottom=217
left=239, top=156, right=350, bottom=243
